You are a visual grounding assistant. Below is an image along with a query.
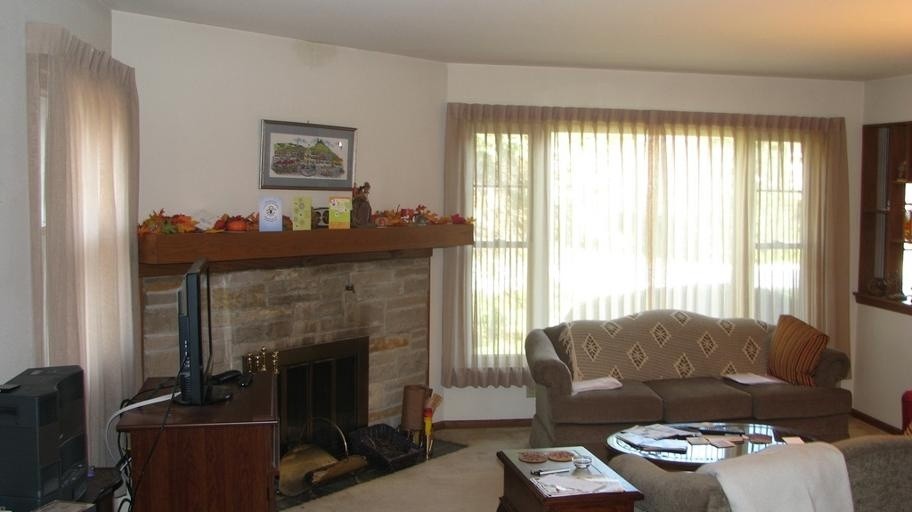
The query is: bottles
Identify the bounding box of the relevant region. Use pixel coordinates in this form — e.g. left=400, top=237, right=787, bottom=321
left=866, top=276, right=889, bottom=296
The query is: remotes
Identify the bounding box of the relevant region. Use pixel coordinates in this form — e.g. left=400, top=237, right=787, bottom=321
left=213, top=370, right=240, bottom=382
left=239, top=373, right=253, bottom=387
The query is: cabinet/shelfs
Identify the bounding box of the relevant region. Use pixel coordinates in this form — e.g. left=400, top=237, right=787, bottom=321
left=852, top=122, right=912, bottom=315
left=102, top=369, right=281, bottom=512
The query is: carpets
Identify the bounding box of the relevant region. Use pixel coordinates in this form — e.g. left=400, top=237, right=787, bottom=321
left=277, top=424, right=468, bottom=512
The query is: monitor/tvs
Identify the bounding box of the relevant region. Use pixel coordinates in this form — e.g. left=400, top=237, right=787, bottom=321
left=171, top=257, right=233, bottom=407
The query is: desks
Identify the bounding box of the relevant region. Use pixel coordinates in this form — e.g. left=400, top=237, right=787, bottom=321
left=61, top=467, right=124, bottom=511
left=493, top=444, right=645, bottom=512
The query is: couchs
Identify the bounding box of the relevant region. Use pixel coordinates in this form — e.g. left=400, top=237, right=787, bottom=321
left=516, top=306, right=853, bottom=449
left=603, top=422, right=911, bottom=510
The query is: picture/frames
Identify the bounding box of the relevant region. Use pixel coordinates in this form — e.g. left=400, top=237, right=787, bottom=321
left=260, top=118, right=357, bottom=192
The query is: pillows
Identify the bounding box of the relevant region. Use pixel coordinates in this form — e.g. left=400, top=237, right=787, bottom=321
left=766, top=313, right=831, bottom=389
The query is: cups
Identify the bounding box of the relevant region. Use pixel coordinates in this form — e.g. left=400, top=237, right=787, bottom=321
left=570, top=450, right=592, bottom=470
